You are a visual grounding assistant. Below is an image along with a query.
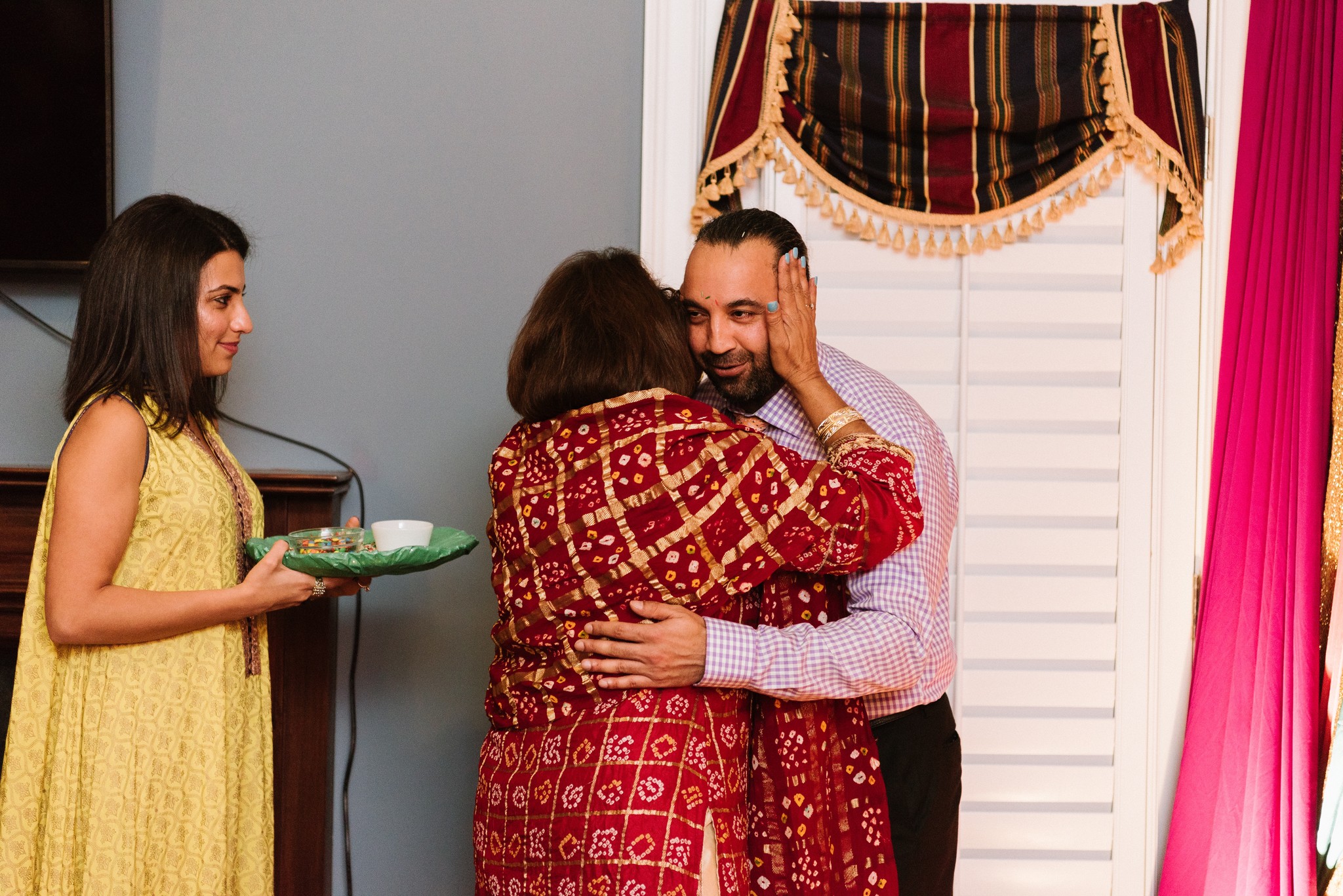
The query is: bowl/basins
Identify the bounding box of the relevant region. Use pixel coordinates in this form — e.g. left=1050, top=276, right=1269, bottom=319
left=289, top=526, right=366, bottom=554
left=370, top=519, right=434, bottom=552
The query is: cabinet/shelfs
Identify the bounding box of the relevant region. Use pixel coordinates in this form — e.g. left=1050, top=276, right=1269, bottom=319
left=625, top=0, right=1251, bottom=896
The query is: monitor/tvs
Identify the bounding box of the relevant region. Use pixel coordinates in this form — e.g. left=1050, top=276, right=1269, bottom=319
left=1, top=0, right=115, bottom=286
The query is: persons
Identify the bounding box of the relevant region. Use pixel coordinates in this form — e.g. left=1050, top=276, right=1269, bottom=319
left=570, top=216, right=969, bottom=896
left=470, top=243, right=927, bottom=896
left=0, top=190, right=371, bottom=896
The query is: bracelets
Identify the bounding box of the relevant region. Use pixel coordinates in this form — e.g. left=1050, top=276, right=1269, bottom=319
left=814, top=405, right=866, bottom=448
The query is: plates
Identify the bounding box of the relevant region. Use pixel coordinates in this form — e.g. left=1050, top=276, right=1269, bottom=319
left=243, top=527, right=480, bottom=577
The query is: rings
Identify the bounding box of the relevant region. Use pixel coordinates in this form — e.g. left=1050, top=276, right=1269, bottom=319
left=312, top=575, right=326, bottom=601
left=356, top=579, right=371, bottom=593
left=804, top=303, right=814, bottom=311
